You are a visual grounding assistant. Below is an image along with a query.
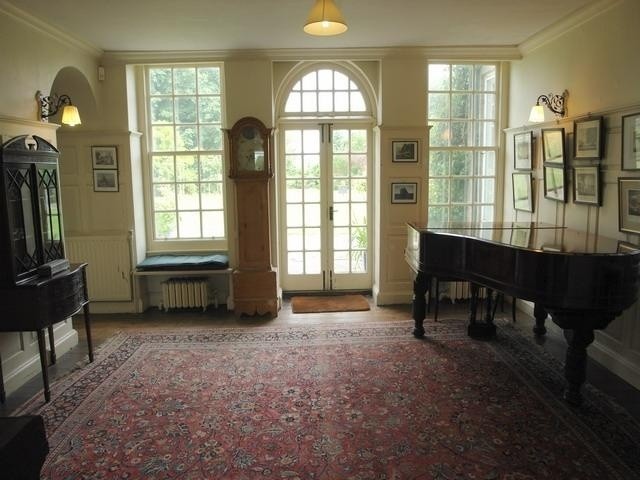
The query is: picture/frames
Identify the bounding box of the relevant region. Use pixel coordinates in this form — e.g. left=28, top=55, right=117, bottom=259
left=509, top=223, right=532, bottom=249
left=511, top=172, right=535, bottom=213
left=542, top=164, right=567, bottom=203
left=390, top=182, right=417, bottom=204
left=541, top=128, right=566, bottom=164
left=514, top=130, right=533, bottom=171
left=616, top=240, right=640, bottom=255
left=572, top=164, right=601, bottom=207
left=91, top=145, right=120, bottom=192
left=391, top=140, right=419, bottom=163
left=617, top=176, right=640, bottom=236
left=573, top=116, right=603, bottom=161
left=621, top=112, right=640, bottom=172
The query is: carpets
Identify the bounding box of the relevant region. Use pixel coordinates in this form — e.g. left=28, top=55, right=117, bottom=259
left=291, top=293, right=371, bottom=314
left=9, top=317, right=639, bottom=480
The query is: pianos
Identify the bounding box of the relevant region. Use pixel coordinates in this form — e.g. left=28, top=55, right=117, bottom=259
left=403, top=221, right=640, bottom=405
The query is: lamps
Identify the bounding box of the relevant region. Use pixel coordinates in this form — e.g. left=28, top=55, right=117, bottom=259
left=528, top=89, right=570, bottom=123
left=301, top=0, right=349, bottom=37
left=35, top=89, right=81, bottom=127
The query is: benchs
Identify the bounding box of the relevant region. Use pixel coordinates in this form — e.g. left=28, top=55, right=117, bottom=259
left=427, top=274, right=517, bottom=324
left=130, top=255, right=234, bottom=276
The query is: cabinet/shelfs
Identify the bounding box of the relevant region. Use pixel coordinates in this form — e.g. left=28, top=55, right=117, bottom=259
left=1, top=133, right=94, bottom=407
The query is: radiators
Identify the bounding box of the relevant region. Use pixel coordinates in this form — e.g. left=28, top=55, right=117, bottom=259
left=160, top=278, right=208, bottom=313
left=440, top=281, right=488, bottom=304
left=65, top=230, right=133, bottom=303
